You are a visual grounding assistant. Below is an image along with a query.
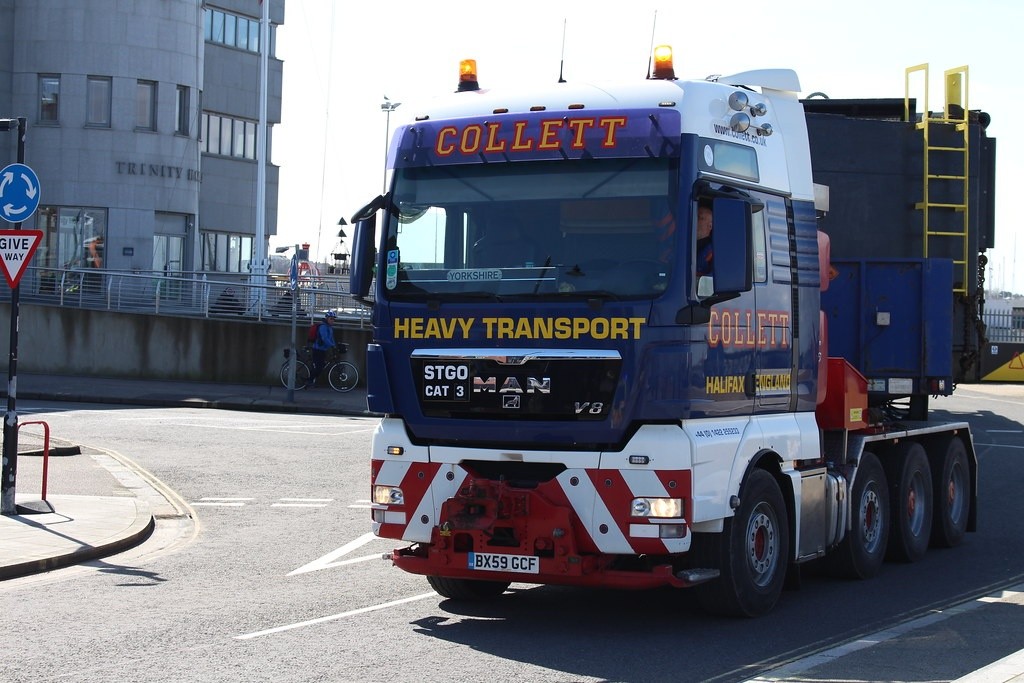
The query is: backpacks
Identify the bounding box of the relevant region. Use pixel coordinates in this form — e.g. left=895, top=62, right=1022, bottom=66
left=308, top=324, right=325, bottom=342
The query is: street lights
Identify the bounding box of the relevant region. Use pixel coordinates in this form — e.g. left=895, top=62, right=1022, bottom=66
left=0, top=116, right=26, bottom=516
left=275, top=244, right=300, bottom=402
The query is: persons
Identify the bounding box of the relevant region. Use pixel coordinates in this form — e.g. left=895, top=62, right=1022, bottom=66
left=646, top=204, right=722, bottom=300
left=304, top=311, right=341, bottom=388
left=212, top=287, right=245, bottom=314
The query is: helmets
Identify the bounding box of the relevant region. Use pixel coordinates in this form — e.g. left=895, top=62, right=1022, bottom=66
left=326, top=312, right=337, bottom=317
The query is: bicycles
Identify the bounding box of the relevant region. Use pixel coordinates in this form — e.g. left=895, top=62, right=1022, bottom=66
left=280, top=342, right=360, bottom=392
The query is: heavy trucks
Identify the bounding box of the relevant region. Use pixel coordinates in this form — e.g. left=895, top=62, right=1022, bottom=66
left=349, top=44, right=997, bottom=621
